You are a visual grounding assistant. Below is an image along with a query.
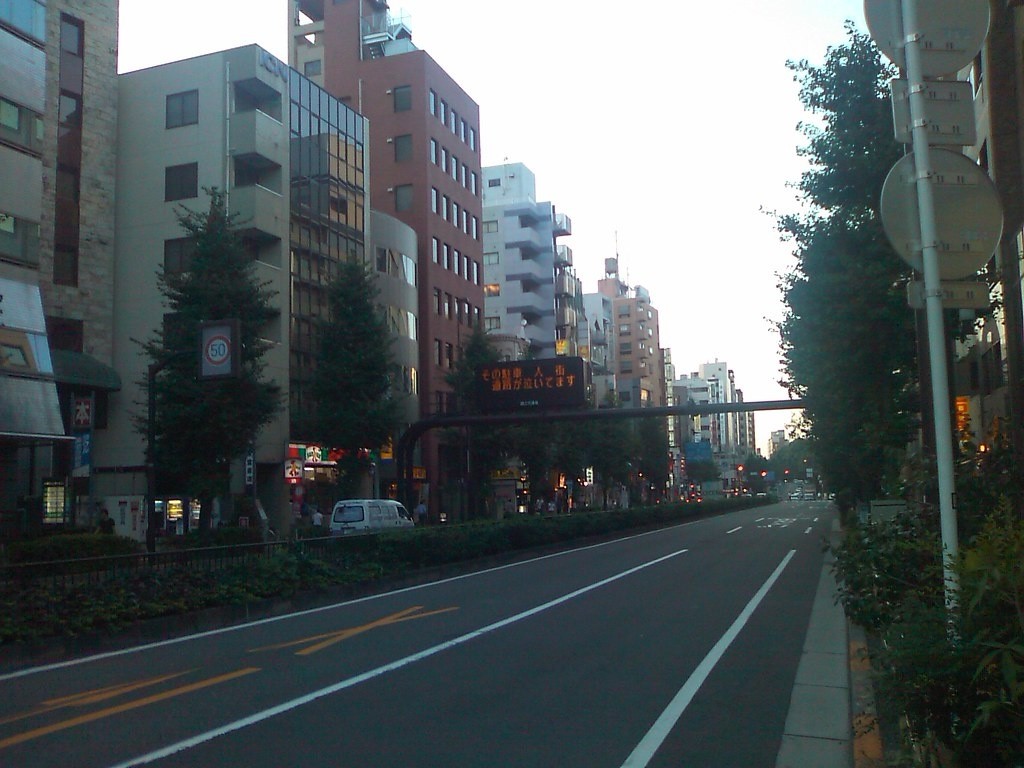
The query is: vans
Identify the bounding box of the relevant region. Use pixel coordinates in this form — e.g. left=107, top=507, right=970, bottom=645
left=328, top=499, right=415, bottom=535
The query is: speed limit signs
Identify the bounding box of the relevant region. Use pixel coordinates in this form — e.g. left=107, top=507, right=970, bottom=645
left=206, top=335, right=231, bottom=365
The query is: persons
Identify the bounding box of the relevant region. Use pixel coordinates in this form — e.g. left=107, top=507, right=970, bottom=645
left=414, top=498, right=428, bottom=526
left=94, top=508, right=117, bottom=536
left=311, top=509, right=323, bottom=528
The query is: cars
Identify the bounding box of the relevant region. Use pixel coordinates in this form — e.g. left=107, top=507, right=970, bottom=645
left=790, top=484, right=816, bottom=501
left=828, top=493, right=835, bottom=500
